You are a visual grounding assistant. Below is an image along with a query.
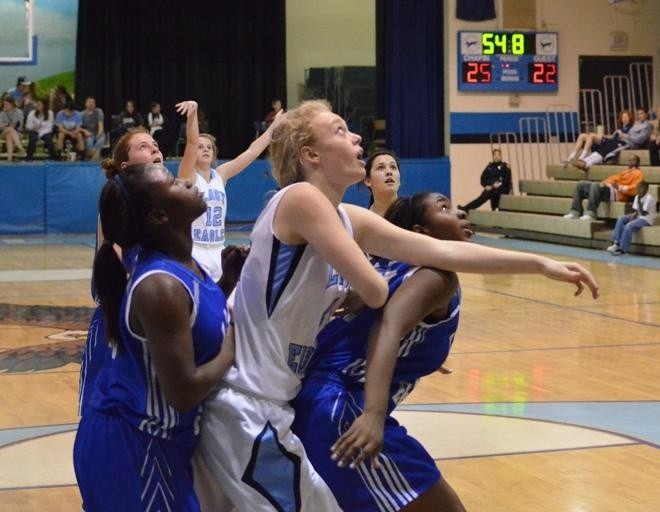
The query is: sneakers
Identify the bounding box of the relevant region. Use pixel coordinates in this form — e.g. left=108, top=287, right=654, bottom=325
left=564, top=213, right=575, bottom=219
left=607, top=244, right=621, bottom=255
left=580, top=215, right=596, bottom=221
left=572, top=160, right=589, bottom=172
left=458, top=205, right=469, bottom=214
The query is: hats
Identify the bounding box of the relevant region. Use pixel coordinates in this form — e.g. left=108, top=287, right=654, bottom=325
left=18, top=77, right=32, bottom=86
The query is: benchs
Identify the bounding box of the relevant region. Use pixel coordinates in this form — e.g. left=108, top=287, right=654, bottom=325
left=468, top=146, right=660, bottom=254
left=0, top=129, right=111, bottom=161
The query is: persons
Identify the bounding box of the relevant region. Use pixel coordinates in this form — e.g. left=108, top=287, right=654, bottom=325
left=191, top=96, right=603, bottom=511
left=72, top=162, right=250, bottom=512
left=0, top=71, right=168, bottom=164
left=457, top=146, right=512, bottom=215
left=172, top=99, right=292, bottom=285
left=363, top=150, right=402, bottom=219
left=261, top=100, right=285, bottom=133
left=288, top=191, right=472, bottom=511
left=91, top=122, right=167, bottom=310
left=559, top=103, right=659, bottom=256
